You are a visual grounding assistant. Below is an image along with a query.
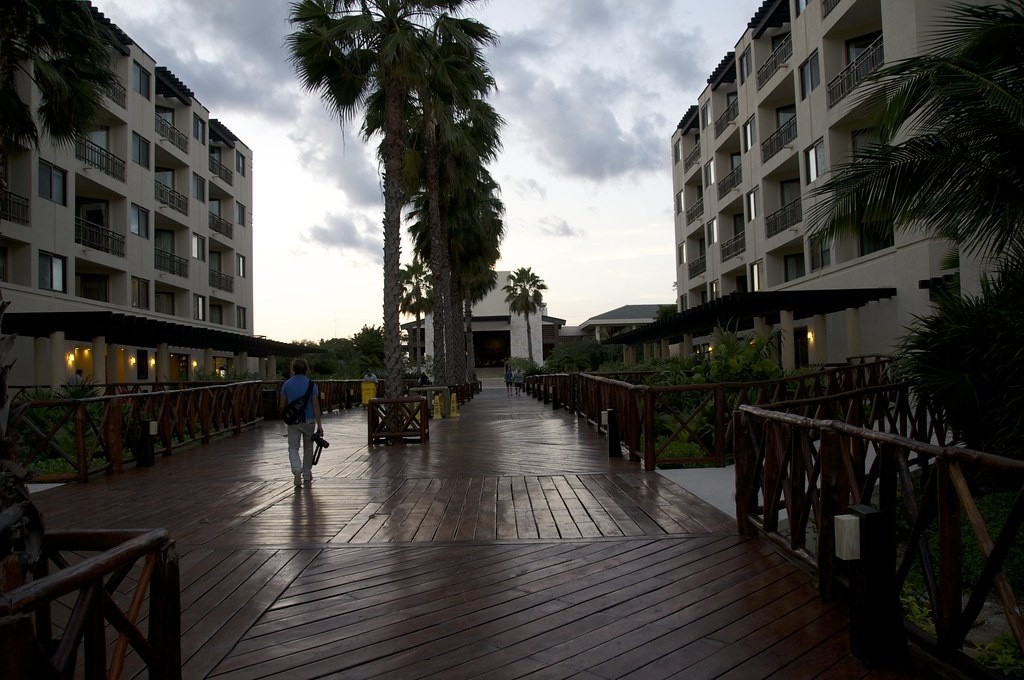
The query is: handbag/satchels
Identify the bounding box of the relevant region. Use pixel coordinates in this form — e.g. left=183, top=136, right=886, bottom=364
left=281, top=378, right=314, bottom=426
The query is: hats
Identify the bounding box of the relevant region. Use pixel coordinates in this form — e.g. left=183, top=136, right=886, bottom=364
left=367, top=369, right=372, bottom=372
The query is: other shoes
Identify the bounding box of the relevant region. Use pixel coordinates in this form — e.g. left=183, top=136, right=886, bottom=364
left=294, top=472, right=302, bottom=486
left=303, top=475, right=312, bottom=481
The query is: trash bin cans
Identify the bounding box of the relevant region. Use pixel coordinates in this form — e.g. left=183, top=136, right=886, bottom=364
left=360, top=381, right=377, bottom=409
left=261, top=389, right=278, bottom=420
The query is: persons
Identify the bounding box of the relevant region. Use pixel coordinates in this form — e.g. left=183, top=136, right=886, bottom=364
left=513, top=368, right=524, bottom=396
left=420, top=372, right=429, bottom=387
left=503, top=366, right=513, bottom=396
left=279, top=358, right=324, bottom=487
left=363, top=369, right=378, bottom=384
left=68, top=369, right=83, bottom=385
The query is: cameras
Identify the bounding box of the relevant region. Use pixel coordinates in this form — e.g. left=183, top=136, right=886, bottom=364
left=311, top=431, right=329, bottom=448
